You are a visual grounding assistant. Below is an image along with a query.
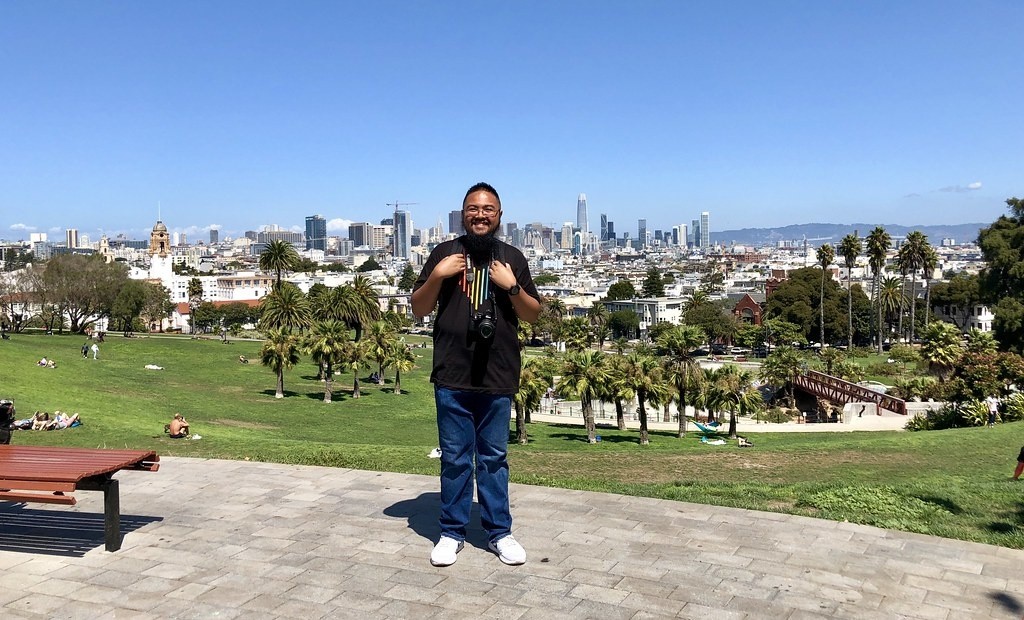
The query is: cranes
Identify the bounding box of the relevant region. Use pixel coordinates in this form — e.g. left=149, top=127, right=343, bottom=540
left=386, top=200, right=417, bottom=211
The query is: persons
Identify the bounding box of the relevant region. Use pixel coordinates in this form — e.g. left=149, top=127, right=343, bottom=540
left=37, top=356, right=56, bottom=368
left=81, top=343, right=89, bottom=359
left=220, top=332, right=224, bottom=341
left=0, top=333, right=10, bottom=340
left=369, top=372, right=379, bottom=383
left=406, top=341, right=426, bottom=351
left=701, top=421, right=754, bottom=447
left=43, top=323, right=49, bottom=335
left=986, top=393, right=997, bottom=428
left=86, top=327, right=106, bottom=342
left=410, top=182, right=541, bottom=567
left=91, top=341, right=99, bottom=360
left=1013, top=446, right=1024, bottom=479
left=551, top=409, right=561, bottom=414
left=124, top=331, right=132, bottom=337
left=0, top=398, right=83, bottom=443
left=144, top=364, right=163, bottom=370
left=239, top=355, right=248, bottom=363
left=170, top=413, right=189, bottom=438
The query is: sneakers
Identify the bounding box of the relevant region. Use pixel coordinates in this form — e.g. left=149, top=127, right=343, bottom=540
left=431, top=535, right=464, bottom=566
left=488, top=535, right=526, bottom=565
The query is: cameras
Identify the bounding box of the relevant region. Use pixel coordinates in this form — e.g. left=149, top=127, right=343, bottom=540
left=469, top=309, right=497, bottom=338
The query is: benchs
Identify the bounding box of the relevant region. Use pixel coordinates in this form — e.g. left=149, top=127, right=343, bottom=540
left=0, top=443, right=160, bottom=553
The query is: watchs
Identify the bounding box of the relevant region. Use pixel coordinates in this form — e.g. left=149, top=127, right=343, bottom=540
left=505, top=282, right=521, bottom=296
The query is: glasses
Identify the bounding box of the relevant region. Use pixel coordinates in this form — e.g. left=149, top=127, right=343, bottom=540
left=464, top=208, right=499, bottom=217
